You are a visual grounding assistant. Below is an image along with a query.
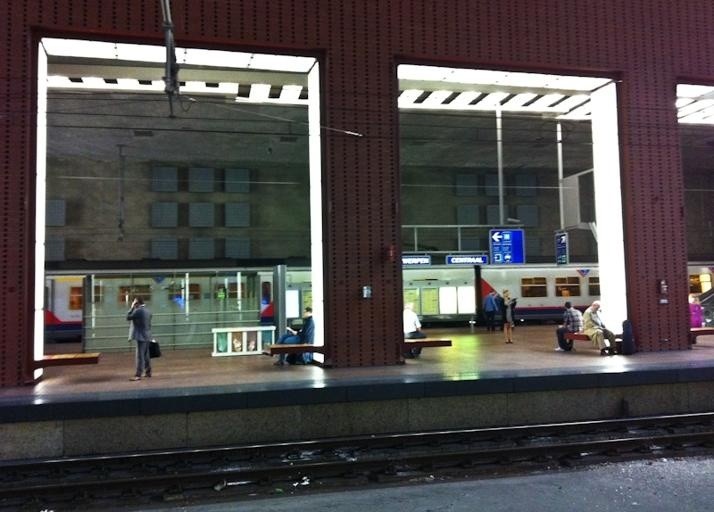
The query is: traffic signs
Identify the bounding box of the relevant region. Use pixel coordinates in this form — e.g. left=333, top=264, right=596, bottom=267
left=488, top=228, right=526, bottom=265
left=554, top=232, right=570, bottom=266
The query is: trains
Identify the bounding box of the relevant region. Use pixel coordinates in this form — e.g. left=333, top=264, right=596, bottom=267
left=45, top=264, right=713, bottom=342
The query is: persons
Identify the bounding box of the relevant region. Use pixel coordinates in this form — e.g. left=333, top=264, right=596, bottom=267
left=582, top=299, right=620, bottom=356
left=689, top=297, right=704, bottom=344
left=125, top=295, right=152, bottom=381
left=403, top=302, right=427, bottom=356
left=493, top=293, right=505, bottom=331
left=482, top=290, right=499, bottom=331
left=262, top=307, right=314, bottom=366
left=501, top=288, right=517, bottom=344
left=553, top=301, right=583, bottom=352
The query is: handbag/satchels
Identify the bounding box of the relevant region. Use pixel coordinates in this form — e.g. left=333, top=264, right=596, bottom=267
left=149, top=341, right=161, bottom=359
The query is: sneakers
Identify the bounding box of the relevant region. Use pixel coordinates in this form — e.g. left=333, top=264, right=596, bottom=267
left=601, top=348, right=617, bottom=355
left=555, top=346, right=564, bottom=352
left=129, top=375, right=149, bottom=381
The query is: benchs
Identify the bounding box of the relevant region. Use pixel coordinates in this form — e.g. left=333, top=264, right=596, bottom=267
left=35, top=353, right=100, bottom=368
left=266, top=345, right=324, bottom=355
left=403, top=339, right=452, bottom=347
left=565, top=333, right=624, bottom=340
left=690, top=329, right=714, bottom=336
